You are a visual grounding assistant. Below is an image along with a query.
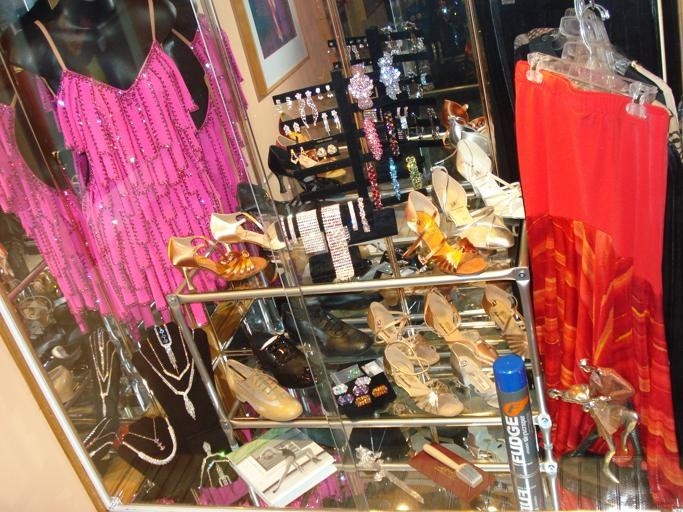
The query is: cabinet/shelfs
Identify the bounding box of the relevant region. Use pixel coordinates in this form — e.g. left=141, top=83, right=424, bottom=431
left=163, top=183, right=562, bottom=512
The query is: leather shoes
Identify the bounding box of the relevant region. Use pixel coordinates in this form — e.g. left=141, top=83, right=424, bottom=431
left=226, top=303, right=370, bottom=422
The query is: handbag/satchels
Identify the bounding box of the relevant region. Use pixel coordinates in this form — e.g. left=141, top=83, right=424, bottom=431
left=227, top=427, right=338, bottom=508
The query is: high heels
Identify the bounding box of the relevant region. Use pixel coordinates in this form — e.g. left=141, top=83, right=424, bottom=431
left=368, top=285, right=529, bottom=417
left=402, top=141, right=526, bottom=274
left=268, top=114, right=345, bottom=192
left=167, top=182, right=303, bottom=293
left=441, top=100, right=492, bottom=156
